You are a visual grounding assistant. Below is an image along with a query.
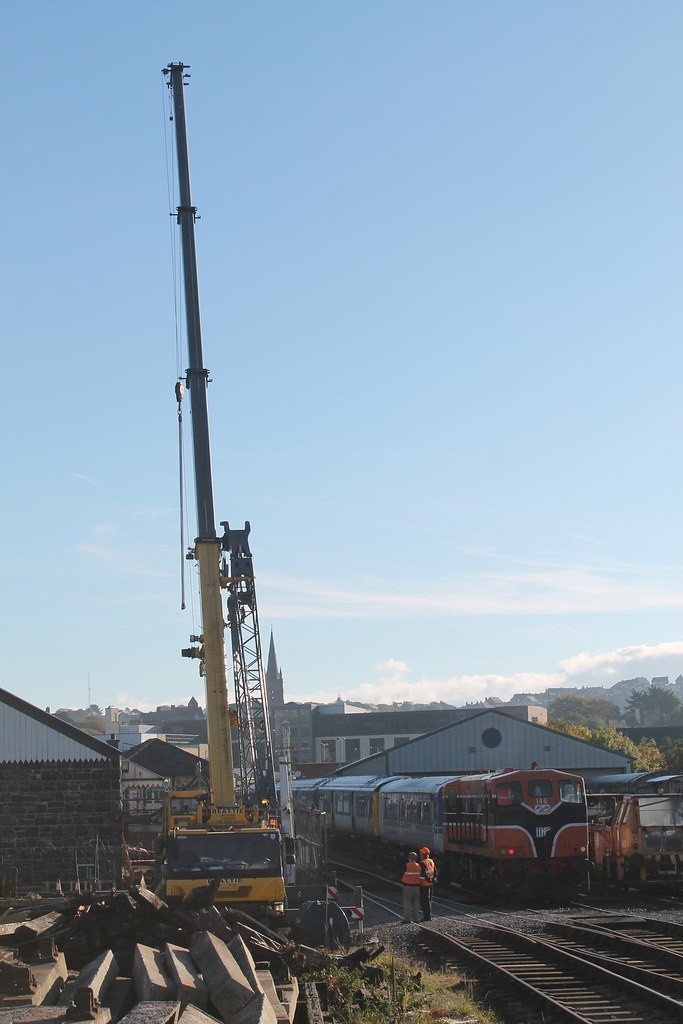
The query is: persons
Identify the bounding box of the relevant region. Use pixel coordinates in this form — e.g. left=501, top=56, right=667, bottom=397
left=418, top=847, right=438, bottom=922
left=399, top=852, right=423, bottom=924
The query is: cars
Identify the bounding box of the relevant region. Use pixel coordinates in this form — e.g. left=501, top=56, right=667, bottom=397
left=128, top=847, right=157, bottom=866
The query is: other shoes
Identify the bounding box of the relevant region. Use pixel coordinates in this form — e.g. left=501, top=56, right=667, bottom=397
left=401, top=920, right=411, bottom=924
left=420, top=918, right=429, bottom=921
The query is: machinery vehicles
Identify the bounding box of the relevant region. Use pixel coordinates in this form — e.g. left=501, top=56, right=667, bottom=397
left=119, top=60, right=380, bottom=945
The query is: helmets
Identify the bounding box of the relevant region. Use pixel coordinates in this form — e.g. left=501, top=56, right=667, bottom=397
left=420, top=847, right=430, bottom=854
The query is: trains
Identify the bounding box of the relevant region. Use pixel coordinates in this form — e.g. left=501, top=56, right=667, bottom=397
left=250, top=768, right=595, bottom=899
left=587, top=792, right=683, bottom=892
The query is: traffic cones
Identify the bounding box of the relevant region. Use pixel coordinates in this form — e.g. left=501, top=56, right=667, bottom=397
left=72, top=879, right=82, bottom=896
left=54, top=878, right=65, bottom=897
